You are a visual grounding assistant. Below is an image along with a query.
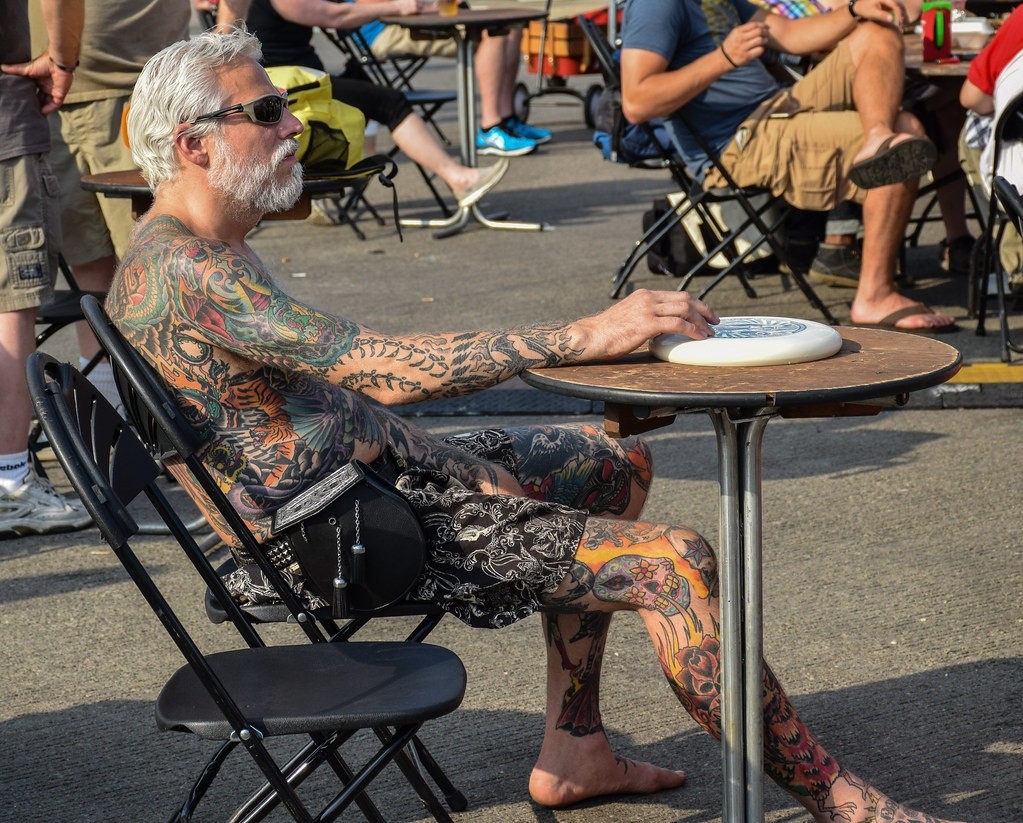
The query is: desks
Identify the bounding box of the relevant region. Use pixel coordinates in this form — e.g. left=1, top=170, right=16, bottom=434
left=78, top=165, right=386, bottom=220
left=379, top=8, right=549, bottom=168
left=519, top=328, right=962, bottom=823
left=899, top=19, right=1009, bottom=299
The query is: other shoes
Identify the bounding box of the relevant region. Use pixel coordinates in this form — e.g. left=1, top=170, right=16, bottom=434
left=453, top=158, right=510, bottom=207
left=939, top=234, right=995, bottom=278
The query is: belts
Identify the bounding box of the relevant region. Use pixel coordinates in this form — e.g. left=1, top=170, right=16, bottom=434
left=231, top=441, right=406, bottom=569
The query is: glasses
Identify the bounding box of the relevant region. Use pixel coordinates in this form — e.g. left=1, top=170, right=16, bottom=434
left=186, top=92, right=290, bottom=126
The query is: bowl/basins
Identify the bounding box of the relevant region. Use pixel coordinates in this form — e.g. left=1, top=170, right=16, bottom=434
left=951, top=17, right=993, bottom=49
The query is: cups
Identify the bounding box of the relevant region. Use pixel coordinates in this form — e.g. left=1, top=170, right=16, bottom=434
left=438, top=0, right=457, bottom=17
left=922, top=0, right=952, bottom=63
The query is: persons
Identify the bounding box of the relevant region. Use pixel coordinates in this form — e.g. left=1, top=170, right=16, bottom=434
left=619, top=0, right=978, bottom=334
left=104, top=20, right=965, bottom=823
left=189, top=0, right=510, bottom=208
left=0, top=0, right=96, bottom=538
left=961, top=3, right=1022, bottom=300
left=350, top=0, right=554, bottom=156
left=31, top=0, right=252, bottom=419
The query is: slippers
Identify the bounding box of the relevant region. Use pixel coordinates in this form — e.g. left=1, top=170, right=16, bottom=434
left=847, top=134, right=938, bottom=190
left=851, top=302, right=954, bottom=333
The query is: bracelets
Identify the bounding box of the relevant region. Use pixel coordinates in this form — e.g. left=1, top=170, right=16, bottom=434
left=719, top=43, right=741, bottom=72
left=847, top=0, right=867, bottom=25
left=48, top=49, right=79, bottom=71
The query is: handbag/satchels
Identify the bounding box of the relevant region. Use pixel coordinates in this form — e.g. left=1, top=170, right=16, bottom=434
left=273, top=458, right=427, bottom=616
left=643, top=186, right=827, bottom=277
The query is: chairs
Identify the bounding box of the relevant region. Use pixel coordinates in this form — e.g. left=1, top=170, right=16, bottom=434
left=79, top=292, right=467, bottom=812
left=577, top=14, right=1023, bottom=363
left=314, top=0, right=458, bottom=243
left=22, top=351, right=468, bottom=823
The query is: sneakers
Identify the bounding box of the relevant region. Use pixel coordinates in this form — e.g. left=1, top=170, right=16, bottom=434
left=809, top=243, right=914, bottom=291
left=506, top=118, right=552, bottom=143
left=476, top=126, right=538, bottom=155
left=0, top=451, right=93, bottom=540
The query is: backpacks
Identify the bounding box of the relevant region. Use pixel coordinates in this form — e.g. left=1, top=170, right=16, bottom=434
left=591, top=50, right=679, bottom=169
left=255, top=62, right=398, bottom=187
